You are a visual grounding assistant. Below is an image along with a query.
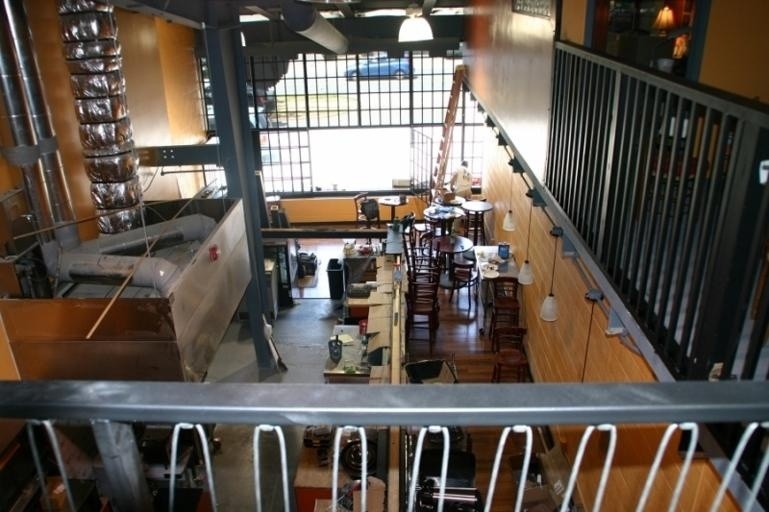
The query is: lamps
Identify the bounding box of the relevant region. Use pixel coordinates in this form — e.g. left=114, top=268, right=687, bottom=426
left=502, top=162, right=573, bottom=326
left=652, top=6, right=674, bottom=37
left=393, top=0, right=440, bottom=45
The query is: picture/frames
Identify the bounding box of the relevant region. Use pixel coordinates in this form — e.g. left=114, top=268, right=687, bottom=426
left=512, top=0, right=555, bottom=21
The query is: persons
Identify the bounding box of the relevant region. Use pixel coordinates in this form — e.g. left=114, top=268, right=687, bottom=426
left=449, top=160, right=475, bottom=202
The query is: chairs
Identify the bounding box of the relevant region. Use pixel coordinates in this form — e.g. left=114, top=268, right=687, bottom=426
left=352, top=185, right=533, bottom=512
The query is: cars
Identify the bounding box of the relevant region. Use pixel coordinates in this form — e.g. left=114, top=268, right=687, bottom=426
left=344, top=54, right=415, bottom=82
left=201, top=73, right=277, bottom=143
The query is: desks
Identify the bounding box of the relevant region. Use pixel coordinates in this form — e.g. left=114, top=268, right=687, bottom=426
left=292, top=451, right=346, bottom=512
left=0, top=422, right=222, bottom=512
left=325, top=322, right=372, bottom=384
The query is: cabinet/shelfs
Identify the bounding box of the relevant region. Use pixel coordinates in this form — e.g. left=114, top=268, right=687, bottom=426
left=236, top=238, right=299, bottom=322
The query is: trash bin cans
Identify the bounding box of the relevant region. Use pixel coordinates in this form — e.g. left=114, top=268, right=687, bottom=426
left=325, top=258, right=344, bottom=299
